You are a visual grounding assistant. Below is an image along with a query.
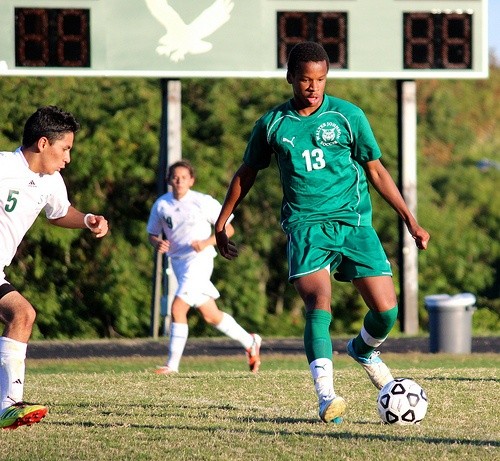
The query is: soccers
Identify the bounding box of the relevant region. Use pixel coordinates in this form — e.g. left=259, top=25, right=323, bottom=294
left=377, top=377, right=429, bottom=426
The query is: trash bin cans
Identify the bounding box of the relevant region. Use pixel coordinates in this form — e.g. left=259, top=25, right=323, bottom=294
left=424, top=293, right=476, bottom=354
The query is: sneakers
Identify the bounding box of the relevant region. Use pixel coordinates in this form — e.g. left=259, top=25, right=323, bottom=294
left=319, top=394, right=347, bottom=423
left=0, top=402, right=48, bottom=430
left=245, top=333, right=262, bottom=373
left=346, top=338, right=394, bottom=390
left=154, top=366, right=174, bottom=375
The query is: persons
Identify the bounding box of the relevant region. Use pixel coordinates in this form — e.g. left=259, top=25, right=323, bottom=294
left=215, top=42, right=431, bottom=424
left=0, top=105, right=108, bottom=434
left=147, top=162, right=261, bottom=374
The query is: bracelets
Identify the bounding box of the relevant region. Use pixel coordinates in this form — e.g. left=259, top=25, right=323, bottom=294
left=84, top=212, right=94, bottom=230
